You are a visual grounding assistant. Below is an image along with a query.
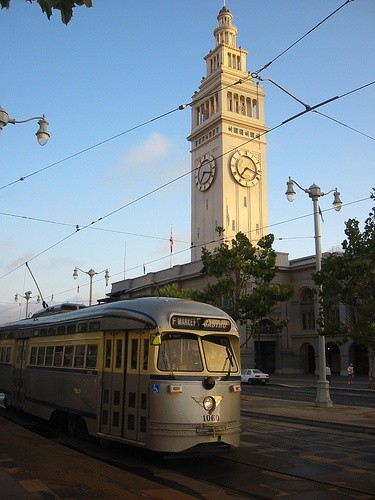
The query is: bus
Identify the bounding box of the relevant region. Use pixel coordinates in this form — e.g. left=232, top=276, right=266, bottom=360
left=1, top=295, right=244, bottom=466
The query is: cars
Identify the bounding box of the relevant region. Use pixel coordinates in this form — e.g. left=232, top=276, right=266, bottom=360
left=240, top=364, right=272, bottom=386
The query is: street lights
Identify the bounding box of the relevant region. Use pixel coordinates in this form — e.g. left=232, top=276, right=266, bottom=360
left=1, top=103, right=52, bottom=148
left=73, top=266, right=110, bottom=306
left=287, top=176, right=343, bottom=410
left=14, top=291, right=40, bottom=318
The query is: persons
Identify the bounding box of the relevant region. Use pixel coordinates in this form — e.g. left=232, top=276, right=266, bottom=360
left=347, top=363, right=355, bottom=385
left=326, top=364, right=330, bottom=381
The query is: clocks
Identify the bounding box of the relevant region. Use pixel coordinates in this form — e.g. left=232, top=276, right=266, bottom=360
left=230, top=150, right=261, bottom=188
left=194, top=154, right=216, bottom=191
